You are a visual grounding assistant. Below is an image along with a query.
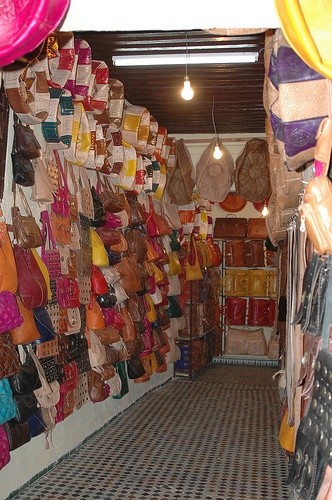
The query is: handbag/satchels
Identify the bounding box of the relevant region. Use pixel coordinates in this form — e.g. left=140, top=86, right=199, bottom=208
left=3, top=113, right=271, bottom=472
left=300, top=118, right=332, bottom=257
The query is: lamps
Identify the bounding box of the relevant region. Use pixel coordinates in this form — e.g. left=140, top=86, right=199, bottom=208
left=112, top=51, right=260, bottom=67
